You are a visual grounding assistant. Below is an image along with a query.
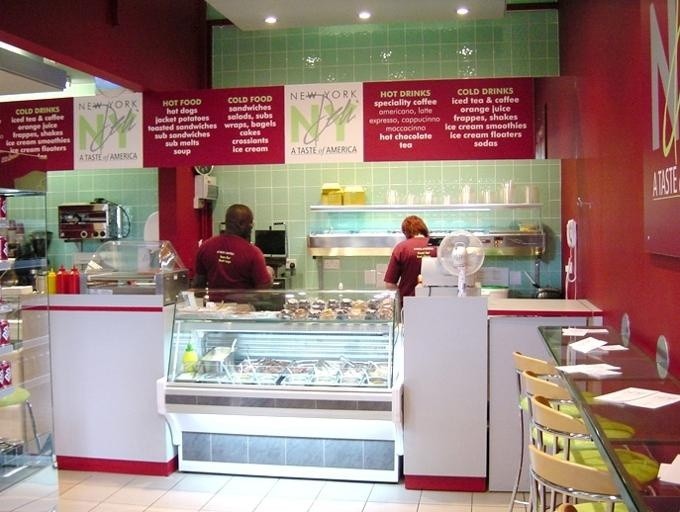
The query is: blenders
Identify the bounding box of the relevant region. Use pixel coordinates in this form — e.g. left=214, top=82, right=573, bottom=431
left=29, top=231, right=53, bottom=257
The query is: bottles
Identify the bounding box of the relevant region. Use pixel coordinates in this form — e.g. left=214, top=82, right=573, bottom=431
left=56, top=266, right=69, bottom=294
left=15, top=223, right=27, bottom=257
left=183, top=345, right=198, bottom=372
left=7, top=220, right=17, bottom=257
left=47, top=267, right=58, bottom=295
left=68, top=267, right=80, bottom=293
left=37, top=271, right=47, bottom=294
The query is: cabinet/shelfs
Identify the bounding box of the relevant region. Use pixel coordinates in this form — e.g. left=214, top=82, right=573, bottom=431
left=0, top=188, right=61, bottom=511
left=157, top=288, right=402, bottom=484
left=307, top=205, right=548, bottom=293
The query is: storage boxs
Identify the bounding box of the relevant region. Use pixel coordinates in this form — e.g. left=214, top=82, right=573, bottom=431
left=320, top=182, right=343, bottom=205
left=342, top=185, right=365, bottom=205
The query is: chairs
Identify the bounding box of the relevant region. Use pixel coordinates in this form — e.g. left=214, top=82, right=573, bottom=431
left=506, top=350, right=660, bottom=511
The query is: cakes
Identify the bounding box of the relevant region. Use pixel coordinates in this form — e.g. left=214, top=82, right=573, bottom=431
left=282, top=296, right=393, bottom=320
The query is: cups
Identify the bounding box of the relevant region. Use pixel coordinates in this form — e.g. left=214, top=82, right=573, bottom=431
left=461, top=186, right=471, bottom=204
left=425, top=189, right=432, bottom=204
left=483, top=191, right=493, bottom=203
left=503, top=180, right=513, bottom=204
left=526, top=186, right=537, bottom=202
left=387, top=188, right=398, bottom=205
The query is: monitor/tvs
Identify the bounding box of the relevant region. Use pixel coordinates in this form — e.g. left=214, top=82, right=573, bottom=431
left=254, top=230, right=286, bottom=257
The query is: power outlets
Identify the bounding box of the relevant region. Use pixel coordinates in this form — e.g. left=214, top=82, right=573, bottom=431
left=286, top=259, right=296, bottom=270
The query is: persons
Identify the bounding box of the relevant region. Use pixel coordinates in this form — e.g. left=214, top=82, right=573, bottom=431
left=194, top=204, right=274, bottom=302
left=384, top=216, right=436, bottom=308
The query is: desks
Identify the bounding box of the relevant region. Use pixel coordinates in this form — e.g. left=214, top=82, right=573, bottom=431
left=538, top=325, right=679, bottom=512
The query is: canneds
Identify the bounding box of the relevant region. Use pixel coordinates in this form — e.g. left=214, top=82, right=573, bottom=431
left=0, top=193, right=8, bottom=222
left=0, top=235, right=9, bottom=261
left=0, top=362, right=4, bottom=388
left=2, top=360, right=12, bottom=388
left=0, top=444, right=10, bottom=466
left=6, top=438, right=22, bottom=466
left=0, top=319, right=9, bottom=346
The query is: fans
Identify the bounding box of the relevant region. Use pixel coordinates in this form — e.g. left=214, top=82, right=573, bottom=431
left=439, top=230, right=486, bottom=297
